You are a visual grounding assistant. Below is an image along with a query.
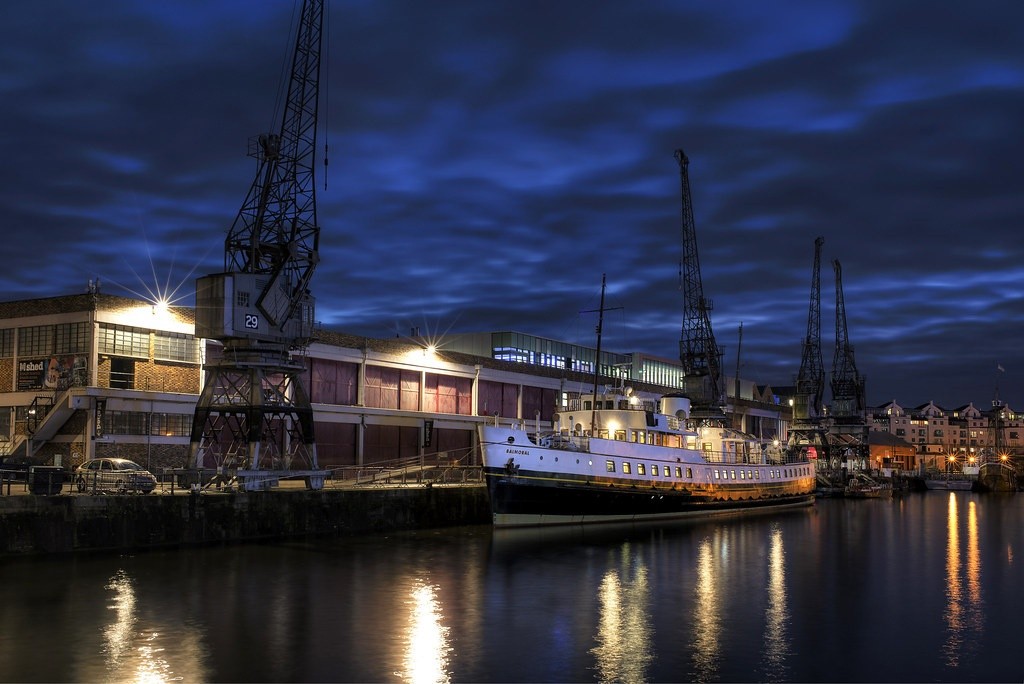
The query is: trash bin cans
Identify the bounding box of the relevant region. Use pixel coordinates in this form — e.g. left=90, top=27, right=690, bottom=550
left=29, top=465, right=63, bottom=496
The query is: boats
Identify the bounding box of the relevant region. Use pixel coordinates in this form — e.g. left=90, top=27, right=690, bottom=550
left=478, top=272, right=816, bottom=540
left=978, top=405, right=1018, bottom=491
left=924, top=473, right=976, bottom=490
left=845, top=473, right=893, bottom=498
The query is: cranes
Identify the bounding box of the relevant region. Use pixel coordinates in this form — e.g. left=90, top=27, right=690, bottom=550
left=180, top=0, right=329, bottom=492
left=672, top=149, right=728, bottom=421
left=827, top=259, right=867, bottom=425
left=793, top=237, right=825, bottom=419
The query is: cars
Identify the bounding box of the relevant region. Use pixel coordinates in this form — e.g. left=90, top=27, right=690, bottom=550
left=74, top=458, right=158, bottom=494
left=0, top=455, right=41, bottom=480
left=227, top=455, right=259, bottom=468
